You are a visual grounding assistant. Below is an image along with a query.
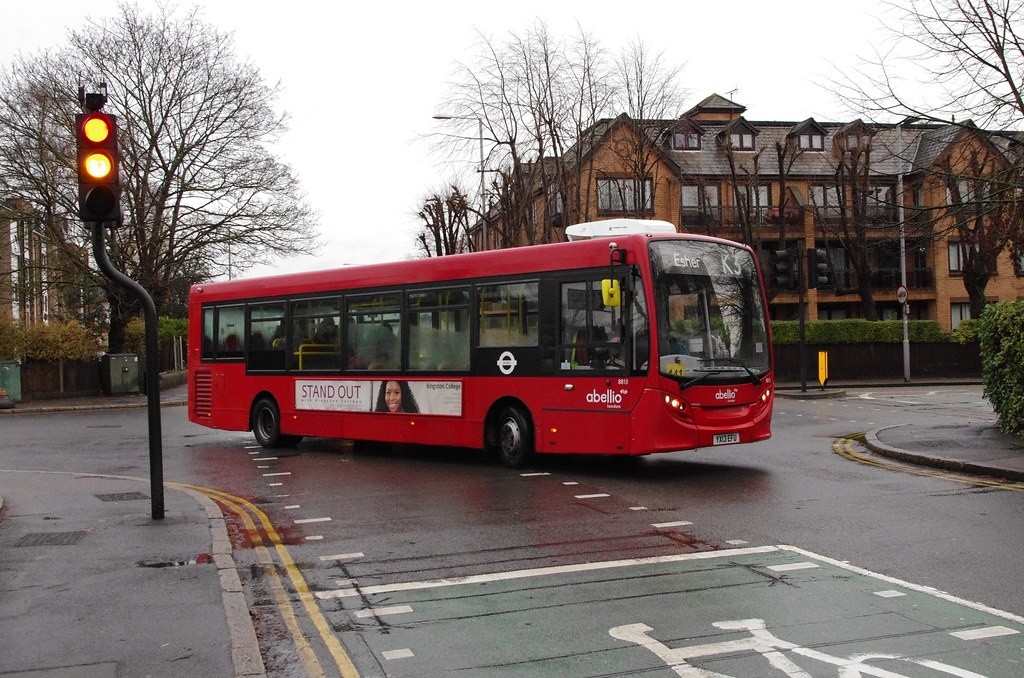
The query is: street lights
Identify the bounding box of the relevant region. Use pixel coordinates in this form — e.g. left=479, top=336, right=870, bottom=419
left=432, top=114, right=487, bottom=251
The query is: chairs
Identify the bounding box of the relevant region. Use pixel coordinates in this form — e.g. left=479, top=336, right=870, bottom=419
left=205, top=316, right=343, bottom=353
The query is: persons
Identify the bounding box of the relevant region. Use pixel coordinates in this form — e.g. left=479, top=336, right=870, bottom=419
left=201, top=316, right=395, bottom=371
left=574, top=326, right=622, bottom=367
left=373, top=380, right=420, bottom=413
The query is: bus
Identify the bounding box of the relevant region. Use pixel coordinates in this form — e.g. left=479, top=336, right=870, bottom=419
left=186, top=215, right=775, bottom=469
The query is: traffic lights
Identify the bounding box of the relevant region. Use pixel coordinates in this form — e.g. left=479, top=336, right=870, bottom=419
left=74, top=112, right=121, bottom=221
left=774, top=245, right=799, bottom=291
left=809, top=246, right=828, bottom=289
